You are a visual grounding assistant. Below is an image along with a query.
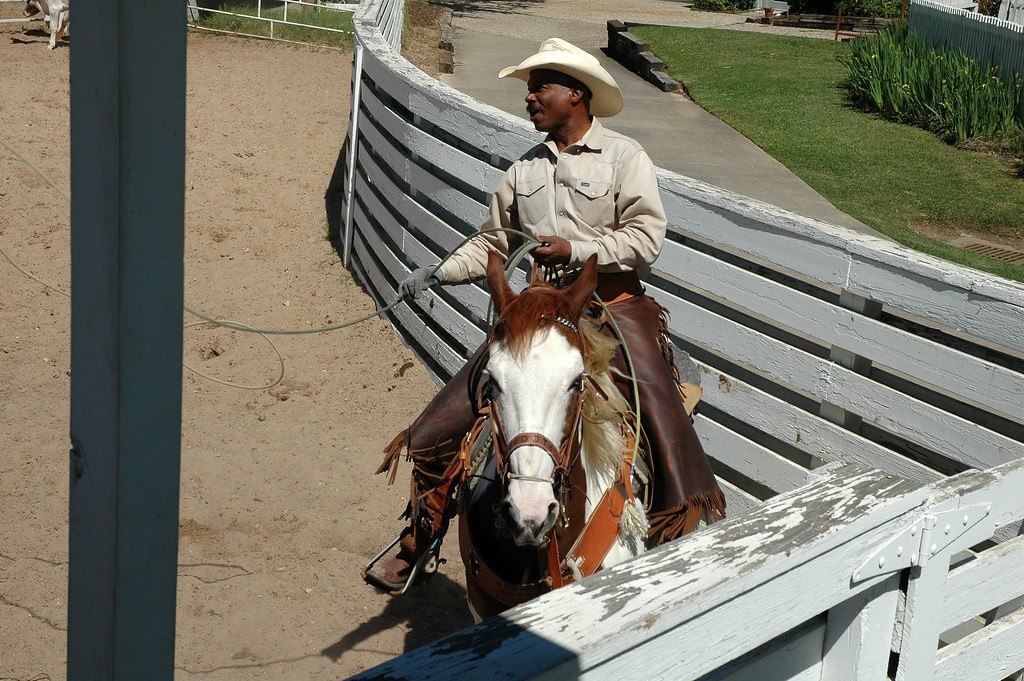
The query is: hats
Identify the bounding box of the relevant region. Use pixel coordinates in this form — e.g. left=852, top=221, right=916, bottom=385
left=498, top=38, right=624, bottom=117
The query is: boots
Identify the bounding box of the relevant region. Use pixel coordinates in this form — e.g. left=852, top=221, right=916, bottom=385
left=366, top=470, right=455, bottom=589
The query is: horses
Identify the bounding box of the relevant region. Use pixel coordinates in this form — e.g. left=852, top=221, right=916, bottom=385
left=459, top=249, right=653, bottom=623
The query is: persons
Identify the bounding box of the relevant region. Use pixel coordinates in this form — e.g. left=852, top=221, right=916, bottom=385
left=366, top=38, right=728, bottom=592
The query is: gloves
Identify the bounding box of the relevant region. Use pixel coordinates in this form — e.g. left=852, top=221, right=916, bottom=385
left=398, top=264, right=445, bottom=298
